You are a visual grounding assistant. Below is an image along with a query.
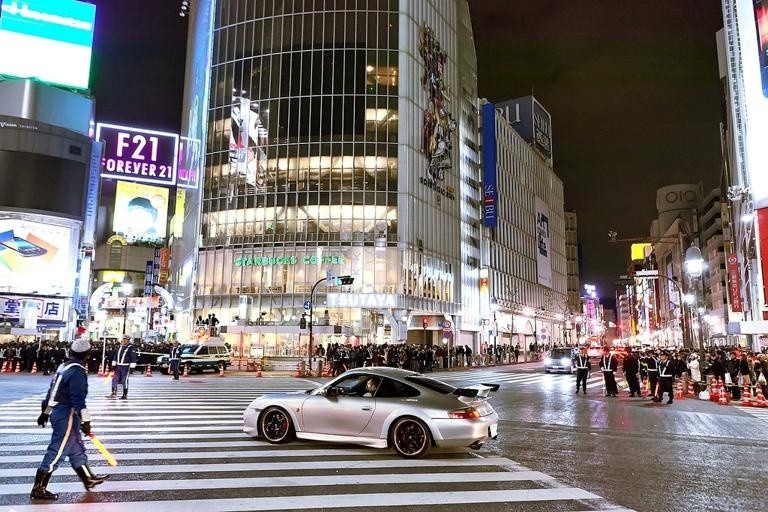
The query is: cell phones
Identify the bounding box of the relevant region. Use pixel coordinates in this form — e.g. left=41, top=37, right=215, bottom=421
left=0, top=237, right=48, bottom=258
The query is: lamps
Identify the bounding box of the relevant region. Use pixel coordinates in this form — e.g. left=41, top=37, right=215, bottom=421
left=684, top=243, right=705, bottom=266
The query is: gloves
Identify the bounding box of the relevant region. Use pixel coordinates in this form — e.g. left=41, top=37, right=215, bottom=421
left=36, top=412, right=49, bottom=428
left=129, top=368, right=135, bottom=375
left=79, top=421, right=91, bottom=437
left=112, top=366, right=118, bottom=371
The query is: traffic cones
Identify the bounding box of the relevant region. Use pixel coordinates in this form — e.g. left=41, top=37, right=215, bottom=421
left=710, top=377, right=718, bottom=402
left=218, top=365, right=224, bottom=377
left=641, top=376, right=649, bottom=397
left=104, top=363, right=110, bottom=377
left=7, top=362, right=14, bottom=373
left=14, top=361, right=22, bottom=374
left=322, top=362, right=327, bottom=377
left=30, top=362, right=37, bottom=375
left=326, top=364, right=331, bottom=376
left=740, top=384, right=753, bottom=406
left=144, top=364, right=152, bottom=377
left=717, top=375, right=730, bottom=401
left=687, top=378, right=695, bottom=396
left=255, top=365, right=263, bottom=378
left=674, top=375, right=687, bottom=401
left=718, top=387, right=729, bottom=406
left=754, top=382, right=768, bottom=408
left=182, top=364, right=189, bottom=377
left=0, top=361, right=7, bottom=373
left=97, top=363, right=103, bottom=378
left=84, top=363, right=89, bottom=374
left=295, top=364, right=300, bottom=378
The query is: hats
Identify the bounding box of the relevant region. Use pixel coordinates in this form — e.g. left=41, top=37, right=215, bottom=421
left=602, top=347, right=610, bottom=351
left=659, top=350, right=668, bottom=355
left=121, top=334, right=130, bottom=339
left=578, top=346, right=586, bottom=350
left=690, top=352, right=697, bottom=358
left=71, top=339, right=91, bottom=354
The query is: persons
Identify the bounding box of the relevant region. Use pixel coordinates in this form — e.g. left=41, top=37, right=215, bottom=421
left=670, top=343, right=768, bottom=402
left=363, top=377, right=379, bottom=397
left=314, top=341, right=590, bottom=377
left=28, top=337, right=111, bottom=501
left=0, top=334, right=182, bottom=382
left=104, top=334, right=138, bottom=401
left=623, top=345, right=675, bottom=405
left=573, top=347, right=591, bottom=393
left=600, top=347, right=619, bottom=397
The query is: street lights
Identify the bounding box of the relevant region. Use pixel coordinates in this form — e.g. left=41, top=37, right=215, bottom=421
left=532, top=312, right=538, bottom=352
left=685, top=242, right=707, bottom=391
left=686, top=291, right=695, bottom=345
left=573, top=314, right=582, bottom=345
left=490, top=298, right=497, bottom=352
left=697, top=301, right=705, bottom=347
left=555, top=312, right=561, bottom=344
left=705, top=313, right=722, bottom=346
left=585, top=318, right=603, bottom=344
left=120, top=270, right=132, bottom=337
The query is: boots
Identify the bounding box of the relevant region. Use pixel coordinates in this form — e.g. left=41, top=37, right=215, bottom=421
left=651, top=396, right=673, bottom=404
left=30, top=469, right=59, bottom=500
left=628, top=390, right=643, bottom=397
left=106, top=387, right=117, bottom=398
left=584, top=391, right=587, bottom=394
left=605, top=391, right=611, bottom=396
left=119, top=389, right=128, bottom=400
left=75, top=463, right=110, bottom=492
left=576, top=389, right=579, bottom=393
left=612, top=391, right=616, bottom=397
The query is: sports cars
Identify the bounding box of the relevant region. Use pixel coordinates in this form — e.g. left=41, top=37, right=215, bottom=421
left=241, top=366, right=501, bottom=460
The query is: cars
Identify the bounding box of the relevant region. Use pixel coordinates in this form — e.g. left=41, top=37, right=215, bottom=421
left=631, top=345, right=646, bottom=353
left=574, top=345, right=587, bottom=355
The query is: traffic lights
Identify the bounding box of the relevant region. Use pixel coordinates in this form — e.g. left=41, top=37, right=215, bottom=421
left=422, top=318, right=427, bottom=329
left=337, top=277, right=354, bottom=285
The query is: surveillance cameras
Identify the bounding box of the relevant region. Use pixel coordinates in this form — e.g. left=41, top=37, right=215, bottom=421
left=608, top=230, right=617, bottom=240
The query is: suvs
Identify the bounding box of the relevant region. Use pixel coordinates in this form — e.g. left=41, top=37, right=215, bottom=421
left=588, top=347, right=605, bottom=358
left=642, top=343, right=651, bottom=351
left=545, top=347, right=579, bottom=374
left=609, top=346, right=630, bottom=357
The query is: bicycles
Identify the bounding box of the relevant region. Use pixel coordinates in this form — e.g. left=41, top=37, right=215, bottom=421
left=467, top=351, right=495, bottom=366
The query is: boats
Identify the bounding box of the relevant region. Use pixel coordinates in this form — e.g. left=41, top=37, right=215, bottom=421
left=299, top=318, right=306, bottom=330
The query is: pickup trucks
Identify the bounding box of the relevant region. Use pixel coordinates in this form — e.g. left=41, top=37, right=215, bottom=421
left=156, top=341, right=231, bottom=375
